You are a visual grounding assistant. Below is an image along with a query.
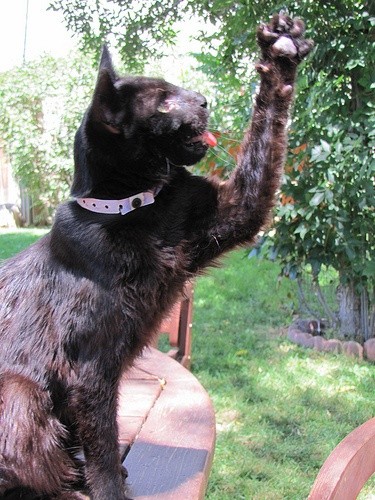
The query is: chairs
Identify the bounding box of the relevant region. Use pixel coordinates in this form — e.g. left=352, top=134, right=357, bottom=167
left=306, top=419, right=375, bottom=500
left=159, top=280, right=194, bottom=366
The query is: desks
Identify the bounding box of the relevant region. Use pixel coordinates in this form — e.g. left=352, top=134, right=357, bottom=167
left=75, top=341, right=216, bottom=499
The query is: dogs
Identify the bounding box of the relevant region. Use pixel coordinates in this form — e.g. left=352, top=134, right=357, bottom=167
left=1, top=9, right=316, bottom=500
